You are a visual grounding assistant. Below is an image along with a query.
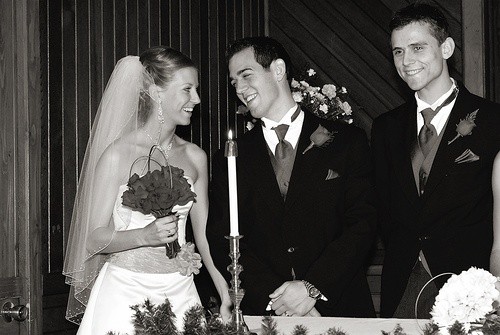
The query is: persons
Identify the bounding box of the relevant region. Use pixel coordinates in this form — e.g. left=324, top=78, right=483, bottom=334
left=208, top=35, right=376, bottom=319
left=62, top=46, right=230, bottom=335
left=370, top=5, right=500, bottom=319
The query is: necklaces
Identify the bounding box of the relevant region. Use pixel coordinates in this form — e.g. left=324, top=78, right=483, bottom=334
left=143, top=127, right=175, bottom=159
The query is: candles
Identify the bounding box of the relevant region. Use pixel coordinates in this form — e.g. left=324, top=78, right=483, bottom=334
left=224, top=130, right=239, bottom=237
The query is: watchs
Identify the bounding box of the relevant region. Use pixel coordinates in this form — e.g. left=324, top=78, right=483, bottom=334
left=300, top=279, right=328, bottom=301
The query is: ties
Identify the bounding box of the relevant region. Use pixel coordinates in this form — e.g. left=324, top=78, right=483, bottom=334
left=419, top=88, right=459, bottom=159
left=271, top=105, right=302, bottom=163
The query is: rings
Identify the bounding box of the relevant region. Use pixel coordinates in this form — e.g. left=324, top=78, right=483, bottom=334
left=284, top=311, right=292, bottom=317
left=167, top=230, right=171, bottom=236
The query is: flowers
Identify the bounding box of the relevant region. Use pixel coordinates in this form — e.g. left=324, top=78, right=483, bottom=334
left=121, top=165, right=197, bottom=259
left=302, top=124, right=339, bottom=155
left=176, top=242, right=202, bottom=277
left=448, top=109, right=480, bottom=145
left=237, top=69, right=363, bottom=131
left=429, top=265, right=500, bottom=335
